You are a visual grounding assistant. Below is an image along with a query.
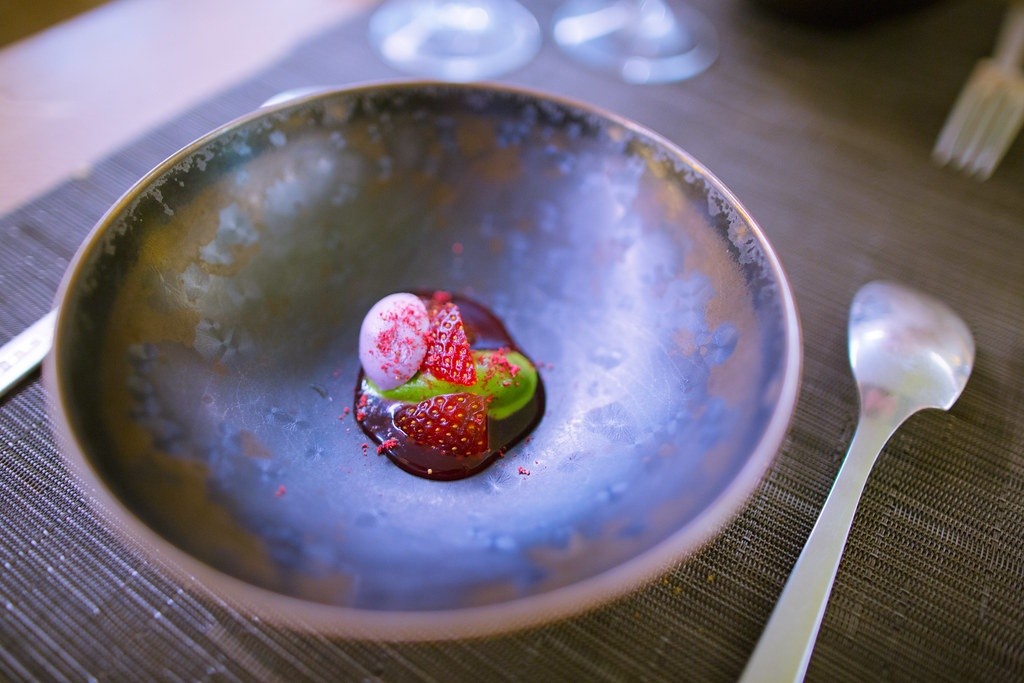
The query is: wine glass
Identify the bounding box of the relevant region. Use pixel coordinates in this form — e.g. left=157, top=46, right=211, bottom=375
left=372, top=0, right=542, bottom=81
left=554, top=0, right=721, bottom=85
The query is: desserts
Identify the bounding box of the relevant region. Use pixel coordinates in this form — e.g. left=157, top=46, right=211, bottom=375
left=357, top=289, right=545, bottom=481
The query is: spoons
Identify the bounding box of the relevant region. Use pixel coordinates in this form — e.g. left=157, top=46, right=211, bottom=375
left=742, top=279, right=975, bottom=683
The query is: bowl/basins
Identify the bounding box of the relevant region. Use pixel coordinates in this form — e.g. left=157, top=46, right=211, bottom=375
left=45, top=83, right=802, bottom=639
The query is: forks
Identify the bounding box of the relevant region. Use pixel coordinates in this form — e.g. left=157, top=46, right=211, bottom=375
left=934, top=5, right=1024, bottom=179
